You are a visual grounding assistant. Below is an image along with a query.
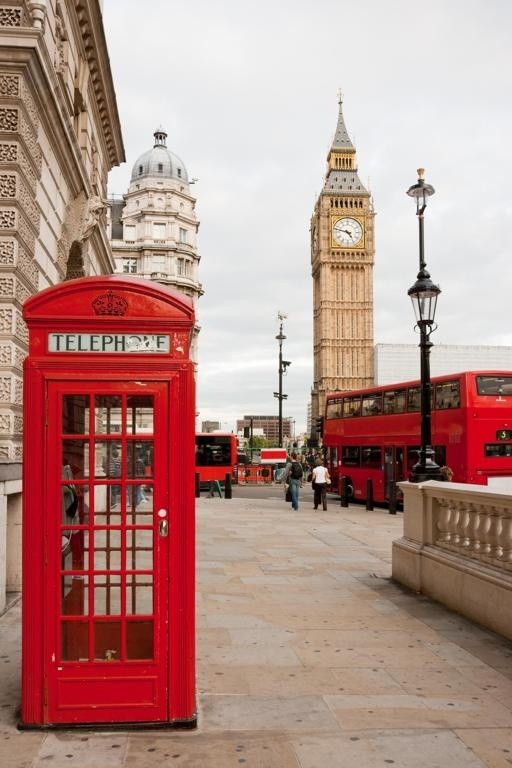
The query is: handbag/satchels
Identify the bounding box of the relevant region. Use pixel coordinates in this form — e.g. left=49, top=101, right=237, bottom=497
left=326, top=476, right=331, bottom=485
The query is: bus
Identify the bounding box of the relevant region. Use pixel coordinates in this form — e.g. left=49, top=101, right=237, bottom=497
left=102, top=429, right=239, bottom=489
left=321, top=370, right=511, bottom=509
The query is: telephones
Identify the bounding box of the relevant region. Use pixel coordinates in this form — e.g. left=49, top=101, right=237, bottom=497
left=62, top=465, right=80, bottom=536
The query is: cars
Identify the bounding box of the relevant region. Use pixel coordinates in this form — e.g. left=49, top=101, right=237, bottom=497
left=238, top=447, right=249, bottom=465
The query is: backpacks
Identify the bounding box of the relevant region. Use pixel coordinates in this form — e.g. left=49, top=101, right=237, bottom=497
left=289, top=462, right=302, bottom=478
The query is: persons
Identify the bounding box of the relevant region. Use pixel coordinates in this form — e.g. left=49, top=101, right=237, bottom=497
left=126, top=453, right=146, bottom=508
left=104, top=450, right=121, bottom=508
left=288, top=449, right=322, bottom=481
left=311, top=457, right=331, bottom=511
left=280, top=453, right=303, bottom=511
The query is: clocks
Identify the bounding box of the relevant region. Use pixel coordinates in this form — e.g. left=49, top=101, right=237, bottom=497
left=328, top=208, right=367, bottom=252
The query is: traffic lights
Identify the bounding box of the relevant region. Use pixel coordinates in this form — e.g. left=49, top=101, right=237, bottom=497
left=315, top=415, right=323, bottom=438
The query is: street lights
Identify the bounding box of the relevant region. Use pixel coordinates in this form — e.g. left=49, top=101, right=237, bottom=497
left=406, top=168, right=444, bottom=482
left=273, top=310, right=293, bottom=446
